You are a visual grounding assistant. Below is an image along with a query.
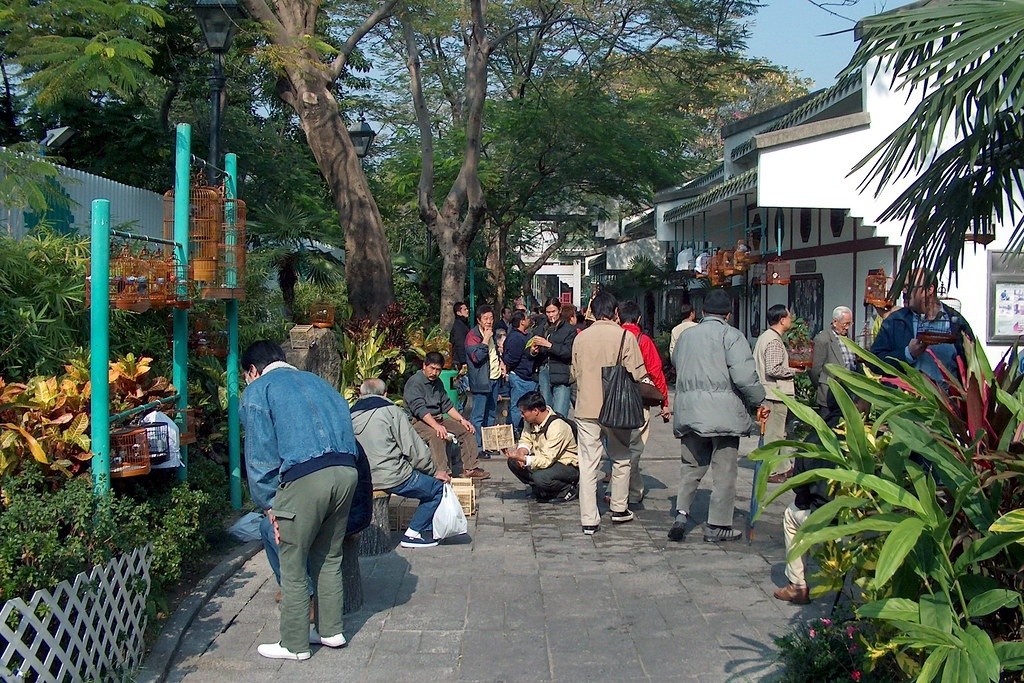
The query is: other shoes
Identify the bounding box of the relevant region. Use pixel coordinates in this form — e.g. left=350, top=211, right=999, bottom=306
left=603, top=469, right=612, bottom=483
left=767, top=465, right=794, bottom=483
left=478, top=449, right=500, bottom=458
left=275, top=589, right=315, bottom=622
left=604, top=491, right=611, bottom=506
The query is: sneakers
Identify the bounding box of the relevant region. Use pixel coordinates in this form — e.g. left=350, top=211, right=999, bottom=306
left=774, top=579, right=810, bottom=603
left=704, top=524, right=742, bottom=542
left=582, top=524, right=600, bottom=534
left=465, top=467, right=490, bottom=479
left=540, top=489, right=576, bottom=503
left=668, top=513, right=688, bottom=541
left=257, top=641, right=310, bottom=660
left=401, top=531, right=438, bottom=548
left=611, top=508, right=634, bottom=522
left=308, top=623, right=346, bottom=647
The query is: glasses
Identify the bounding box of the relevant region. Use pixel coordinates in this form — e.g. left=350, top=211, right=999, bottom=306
left=902, top=284, right=922, bottom=290
left=833, top=317, right=854, bottom=327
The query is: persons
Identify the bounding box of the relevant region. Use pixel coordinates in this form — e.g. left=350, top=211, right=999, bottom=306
left=501, top=391, right=580, bottom=503
left=603, top=302, right=671, bottom=505
left=259, top=438, right=373, bottom=624
left=752, top=304, right=806, bottom=483
left=449, top=298, right=594, bottom=459
left=571, top=293, right=655, bottom=535
left=810, top=306, right=856, bottom=422
left=667, top=289, right=771, bottom=542
left=350, top=378, right=451, bottom=547
left=775, top=376, right=871, bottom=605
left=238, top=340, right=359, bottom=660
left=864, top=267, right=976, bottom=486
left=403, top=352, right=490, bottom=478
left=669, top=304, right=699, bottom=360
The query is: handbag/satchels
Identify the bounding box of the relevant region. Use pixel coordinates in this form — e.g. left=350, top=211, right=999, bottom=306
left=433, top=483, right=467, bottom=540
left=635, top=331, right=663, bottom=406
left=598, top=330, right=645, bottom=429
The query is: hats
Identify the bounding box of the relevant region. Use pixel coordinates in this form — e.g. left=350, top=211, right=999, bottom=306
left=702, top=289, right=732, bottom=315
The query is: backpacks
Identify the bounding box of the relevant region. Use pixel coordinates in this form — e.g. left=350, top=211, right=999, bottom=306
left=530, top=412, right=577, bottom=444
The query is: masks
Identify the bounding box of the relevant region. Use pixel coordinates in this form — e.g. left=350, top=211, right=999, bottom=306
left=245, top=367, right=257, bottom=385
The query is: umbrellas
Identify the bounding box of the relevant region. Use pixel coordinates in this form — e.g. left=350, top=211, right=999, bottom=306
left=749, top=407, right=765, bottom=544
